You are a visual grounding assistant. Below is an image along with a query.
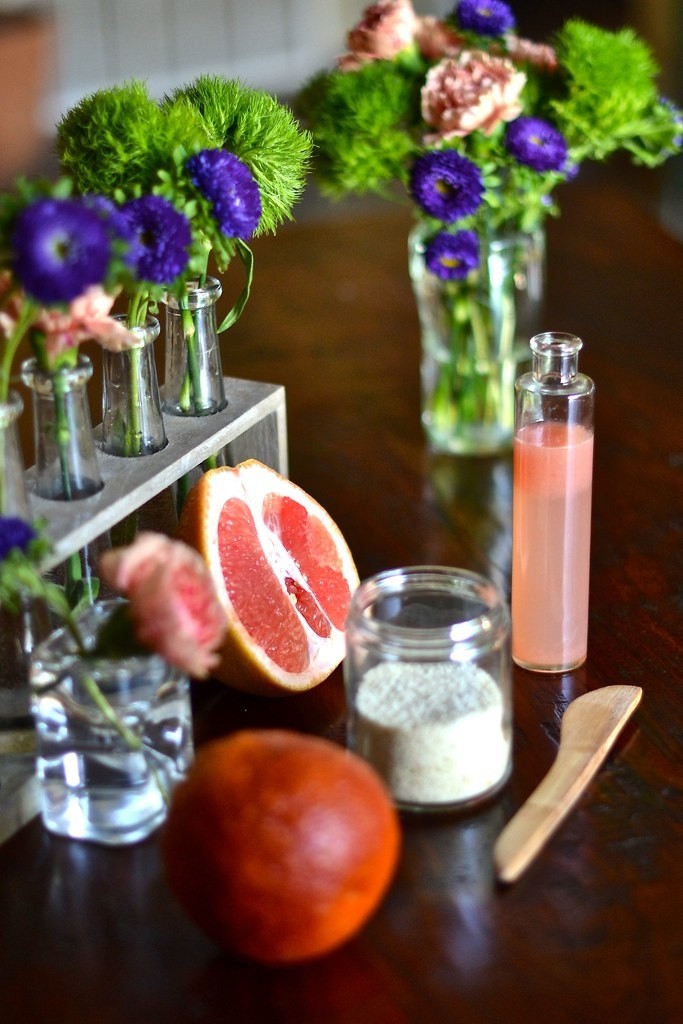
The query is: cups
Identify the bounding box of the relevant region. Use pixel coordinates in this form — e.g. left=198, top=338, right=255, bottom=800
left=29, top=597, right=196, bottom=848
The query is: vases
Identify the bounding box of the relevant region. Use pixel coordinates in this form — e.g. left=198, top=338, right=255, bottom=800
left=491, top=227, right=546, bottom=308
left=20, top=356, right=109, bottom=500
left=21, top=594, right=194, bottom=844
left=0, top=392, right=36, bottom=522
left=156, top=275, right=231, bottom=415
left=409, top=239, right=520, bottom=458
left=99, top=312, right=174, bottom=460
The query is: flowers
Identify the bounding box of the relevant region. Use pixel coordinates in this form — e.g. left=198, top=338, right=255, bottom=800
left=1, top=1, right=683, bottom=496
left=0, top=519, right=226, bottom=750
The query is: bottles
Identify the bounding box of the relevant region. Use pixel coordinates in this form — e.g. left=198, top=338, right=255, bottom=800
left=510, top=330, right=597, bottom=673
left=341, top=563, right=516, bottom=811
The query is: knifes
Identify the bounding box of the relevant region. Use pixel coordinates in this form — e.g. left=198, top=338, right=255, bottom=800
left=492, top=686, right=642, bottom=882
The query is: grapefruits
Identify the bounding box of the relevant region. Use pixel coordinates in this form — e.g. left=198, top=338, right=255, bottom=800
left=176, top=458, right=361, bottom=694
left=160, top=731, right=399, bottom=967
